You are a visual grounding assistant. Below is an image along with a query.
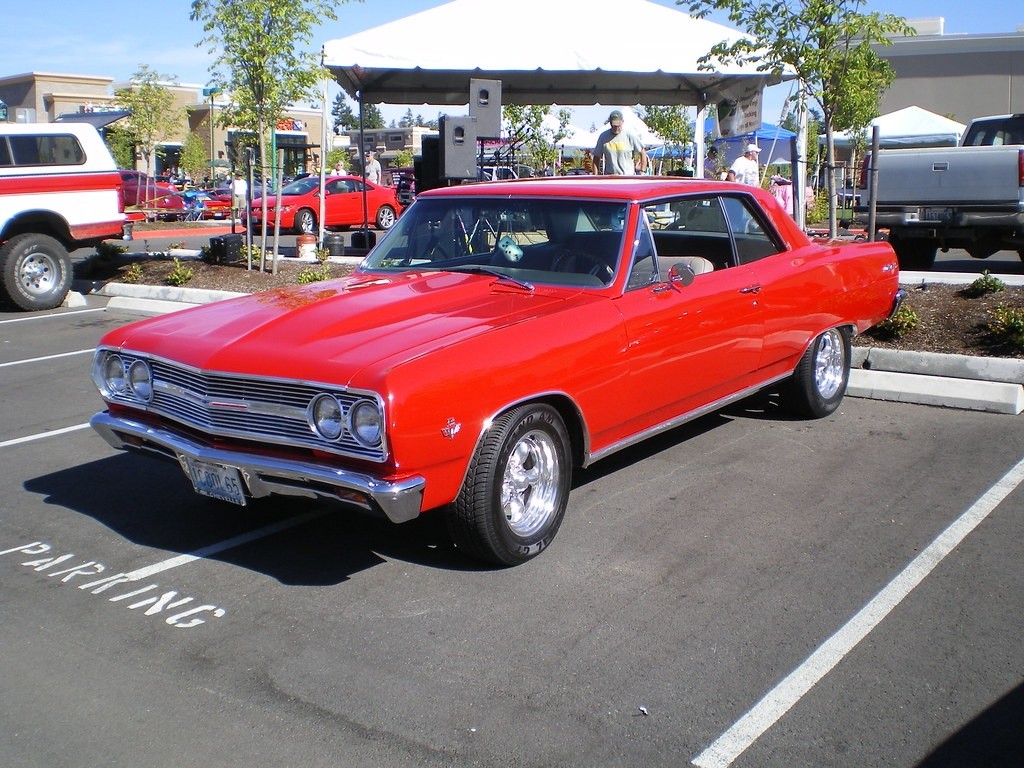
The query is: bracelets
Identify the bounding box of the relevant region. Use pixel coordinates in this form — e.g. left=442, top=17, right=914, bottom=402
left=640, top=169, right=646, bottom=172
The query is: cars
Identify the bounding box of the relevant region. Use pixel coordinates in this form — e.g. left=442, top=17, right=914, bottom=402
left=114, top=167, right=234, bottom=222
left=240, top=173, right=404, bottom=232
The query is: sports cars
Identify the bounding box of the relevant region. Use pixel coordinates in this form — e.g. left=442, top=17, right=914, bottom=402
left=90, top=169, right=907, bottom=568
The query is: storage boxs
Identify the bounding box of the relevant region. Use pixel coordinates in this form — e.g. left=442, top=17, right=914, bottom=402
left=209, top=233, right=244, bottom=263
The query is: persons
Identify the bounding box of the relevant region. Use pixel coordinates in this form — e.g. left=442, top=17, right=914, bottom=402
left=330, top=160, right=345, bottom=181
left=592, top=110, right=647, bottom=177
left=161, top=168, right=209, bottom=191
left=704, top=147, right=721, bottom=179
left=364, top=150, right=381, bottom=185
left=230, top=171, right=248, bottom=217
left=727, top=143, right=763, bottom=187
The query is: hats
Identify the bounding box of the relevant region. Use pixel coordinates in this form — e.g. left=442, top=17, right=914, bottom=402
left=710, top=146, right=718, bottom=155
left=745, top=144, right=762, bottom=152
left=610, top=111, right=623, bottom=126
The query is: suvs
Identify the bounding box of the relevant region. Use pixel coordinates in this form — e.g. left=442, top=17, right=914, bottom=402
left=0, top=107, right=135, bottom=310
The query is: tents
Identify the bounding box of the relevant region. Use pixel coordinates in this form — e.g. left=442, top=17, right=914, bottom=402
left=670, top=115, right=798, bottom=182
left=318, top=0, right=809, bottom=259
left=646, top=143, right=697, bottom=159
left=815, top=105, right=1005, bottom=201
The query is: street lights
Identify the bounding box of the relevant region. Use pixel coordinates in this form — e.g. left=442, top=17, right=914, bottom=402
left=203, top=87, right=225, bottom=186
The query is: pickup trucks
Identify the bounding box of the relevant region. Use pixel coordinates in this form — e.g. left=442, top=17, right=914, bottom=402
left=855, top=110, right=1024, bottom=270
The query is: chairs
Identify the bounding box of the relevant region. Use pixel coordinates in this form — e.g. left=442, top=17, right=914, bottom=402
left=329, top=181, right=340, bottom=194
left=487, top=244, right=584, bottom=274
left=627, top=254, right=717, bottom=291
